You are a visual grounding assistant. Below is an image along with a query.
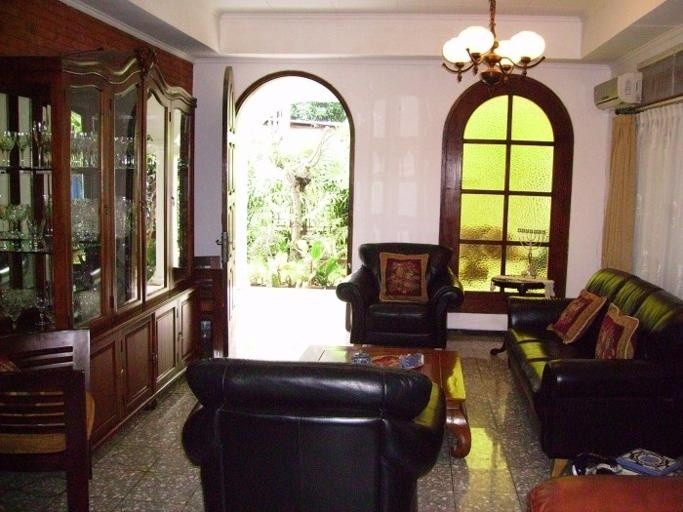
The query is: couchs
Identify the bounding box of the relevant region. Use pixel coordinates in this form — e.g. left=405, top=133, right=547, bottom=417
left=181, top=359, right=447, bottom=512
left=505, top=268, right=683, bottom=459
left=335, top=241, right=463, bottom=350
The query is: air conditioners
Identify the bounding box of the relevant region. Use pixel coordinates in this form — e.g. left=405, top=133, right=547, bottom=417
left=594, top=71, right=644, bottom=111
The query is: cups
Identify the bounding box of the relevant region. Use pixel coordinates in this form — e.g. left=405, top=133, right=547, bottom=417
left=27, top=220, right=46, bottom=247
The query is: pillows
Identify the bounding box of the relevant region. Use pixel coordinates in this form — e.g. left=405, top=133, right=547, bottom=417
left=553, top=288, right=608, bottom=346
left=378, top=252, right=430, bottom=304
left=593, top=301, right=639, bottom=360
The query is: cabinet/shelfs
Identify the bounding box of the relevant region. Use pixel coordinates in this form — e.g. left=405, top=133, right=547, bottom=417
left=91, top=309, right=155, bottom=450
left=144, top=41, right=197, bottom=303
left=156, top=284, right=201, bottom=396
left=1, top=44, right=144, bottom=341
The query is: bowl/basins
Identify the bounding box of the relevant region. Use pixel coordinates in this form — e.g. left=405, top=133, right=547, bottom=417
left=71, top=270, right=90, bottom=290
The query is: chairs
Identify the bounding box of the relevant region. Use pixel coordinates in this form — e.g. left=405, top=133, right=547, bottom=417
left=0, top=329, right=92, bottom=512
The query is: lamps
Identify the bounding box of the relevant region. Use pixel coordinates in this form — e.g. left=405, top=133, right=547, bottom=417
left=442, top=2, right=546, bottom=89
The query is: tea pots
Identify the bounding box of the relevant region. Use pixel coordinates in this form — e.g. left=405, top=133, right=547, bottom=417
left=352, top=351, right=372, bottom=366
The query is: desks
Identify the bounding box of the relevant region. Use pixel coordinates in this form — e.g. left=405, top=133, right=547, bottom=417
left=491, top=275, right=547, bottom=356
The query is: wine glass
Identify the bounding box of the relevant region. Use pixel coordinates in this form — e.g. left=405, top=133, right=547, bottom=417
left=72, top=290, right=102, bottom=323
left=113, top=134, right=134, bottom=167
left=13, top=133, right=30, bottom=169
left=71, top=199, right=101, bottom=248
left=70, top=126, right=100, bottom=167
left=115, top=196, right=130, bottom=238
left=0, top=131, right=15, bottom=169
left=32, top=121, right=46, bottom=169
left=44, top=131, right=54, bottom=168
left=46, top=284, right=55, bottom=325
left=1, top=203, right=28, bottom=240
left=35, top=289, right=49, bottom=325
left=1, top=288, right=36, bottom=331
